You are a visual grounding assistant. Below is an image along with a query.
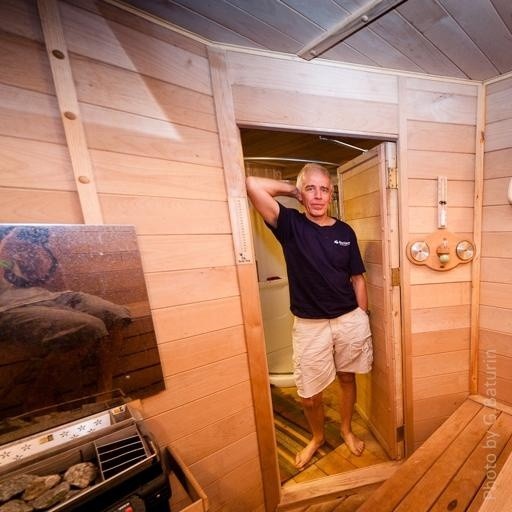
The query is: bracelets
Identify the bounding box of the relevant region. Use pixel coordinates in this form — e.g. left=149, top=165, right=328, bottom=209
left=366, top=310, right=371, bottom=318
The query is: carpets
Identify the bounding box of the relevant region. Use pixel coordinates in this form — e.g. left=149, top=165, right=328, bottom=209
left=270, top=382, right=355, bottom=486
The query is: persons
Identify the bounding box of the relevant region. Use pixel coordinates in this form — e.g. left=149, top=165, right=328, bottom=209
left=0, top=225, right=134, bottom=403
left=245, top=163, right=373, bottom=468
